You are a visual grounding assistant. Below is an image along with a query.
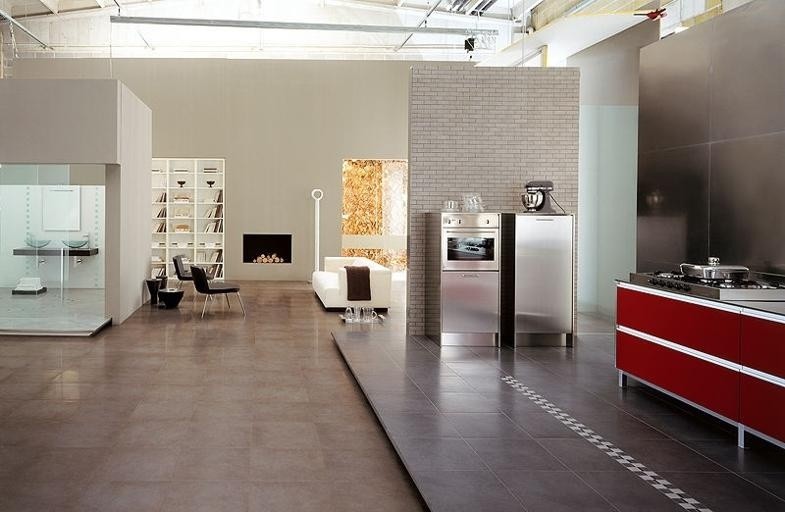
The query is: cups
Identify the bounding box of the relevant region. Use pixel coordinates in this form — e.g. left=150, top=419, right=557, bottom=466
left=443, top=200, right=457, bottom=208
left=363, top=306, right=376, bottom=320
left=345, top=303, right=361, bottom=318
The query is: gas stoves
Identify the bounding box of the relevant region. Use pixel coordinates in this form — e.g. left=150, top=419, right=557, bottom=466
left=628, top=271, right=784, bottom=302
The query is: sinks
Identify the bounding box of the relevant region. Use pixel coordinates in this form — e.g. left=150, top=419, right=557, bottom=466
left=61, top=239, right=87, bottom=248
left=24, top=239, right=50, bottom=248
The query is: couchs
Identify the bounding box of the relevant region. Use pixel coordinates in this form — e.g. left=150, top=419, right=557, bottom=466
left=311, top=256, right=391, bottom=311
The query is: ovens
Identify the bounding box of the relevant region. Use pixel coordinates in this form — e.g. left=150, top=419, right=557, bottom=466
left=443, top=228, right=498, bottom=271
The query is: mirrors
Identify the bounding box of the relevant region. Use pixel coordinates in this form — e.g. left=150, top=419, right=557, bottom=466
left=41, top=186, right=81, bottom=232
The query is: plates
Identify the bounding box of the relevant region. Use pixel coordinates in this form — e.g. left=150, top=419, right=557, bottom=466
left=440, top=209, right=459, bottom=212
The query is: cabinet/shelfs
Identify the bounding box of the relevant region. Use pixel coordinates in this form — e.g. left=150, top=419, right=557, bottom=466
left=615, top=287, right=785, bottom=449
left=151, top=157, right=226, bottom=283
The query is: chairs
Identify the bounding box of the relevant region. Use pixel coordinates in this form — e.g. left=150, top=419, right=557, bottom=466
left=172, top=254, right=246, bottom=319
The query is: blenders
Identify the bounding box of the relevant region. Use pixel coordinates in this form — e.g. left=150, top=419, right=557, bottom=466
left=521, top=181, right=562, bottom=214
left=642, top=190, right=670, bottom=213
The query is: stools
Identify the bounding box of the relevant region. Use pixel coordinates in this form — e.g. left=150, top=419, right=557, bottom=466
left=146, top=276, right=184, bottom=310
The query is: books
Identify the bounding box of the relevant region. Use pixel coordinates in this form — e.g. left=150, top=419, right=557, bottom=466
left=151, top=250, right=223, bottom=278
left=203, top=167, right=217, bottom=171
left=203, top=170, right=217, bottom=173
left=174, top=169, right=188, bottom=173
left=154, top=192, right=166, bottom=234
left=203, top=190, right=223, bottom=232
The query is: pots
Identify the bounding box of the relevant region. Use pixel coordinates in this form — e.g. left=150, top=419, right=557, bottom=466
left=679, top=256, right=749, bottom=283
